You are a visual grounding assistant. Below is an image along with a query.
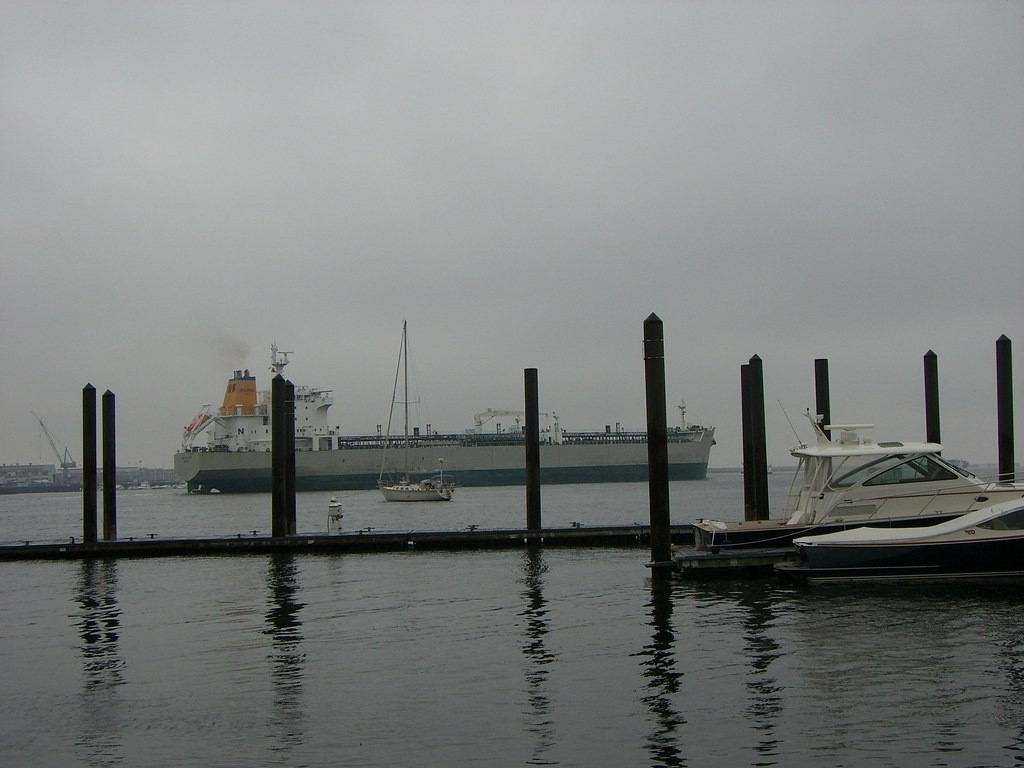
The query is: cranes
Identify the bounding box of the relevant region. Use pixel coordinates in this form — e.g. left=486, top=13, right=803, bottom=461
left=30, top=411, right=77, bottom=469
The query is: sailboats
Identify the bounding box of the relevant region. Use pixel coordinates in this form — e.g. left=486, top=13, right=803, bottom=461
left=377, top=319, right=461, bottom=502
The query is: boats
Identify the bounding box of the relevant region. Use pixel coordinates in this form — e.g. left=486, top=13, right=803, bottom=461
left=643, top=400, right=1024, bottom=580
left=174, top=339, right=717, bottom=494
